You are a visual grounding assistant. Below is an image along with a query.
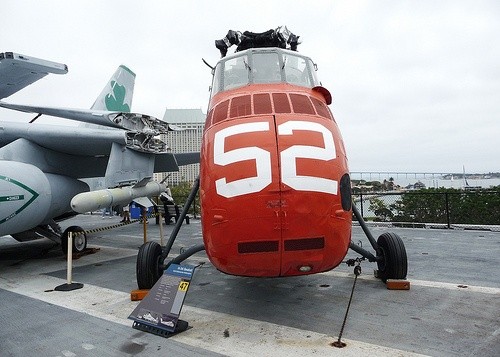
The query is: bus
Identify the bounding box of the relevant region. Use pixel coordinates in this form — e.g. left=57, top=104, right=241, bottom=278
left=463, top=186, right=482, bottom=191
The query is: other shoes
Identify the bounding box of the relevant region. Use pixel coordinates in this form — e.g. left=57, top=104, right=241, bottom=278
left=139, top=221, right=142, bottom=224
left=146, top=222, right=149, bottom=224
left=122, top=220, right=125, bottom=221
left=127, top=220, right=129, bottom=221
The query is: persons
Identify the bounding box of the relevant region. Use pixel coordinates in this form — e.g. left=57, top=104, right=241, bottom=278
left=101, top=204, right=149, bottom=223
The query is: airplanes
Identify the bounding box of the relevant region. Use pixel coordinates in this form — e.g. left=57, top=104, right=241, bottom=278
left=137, top=27, right=408, bottom=288
left=0, top=52, right=200, bottom=254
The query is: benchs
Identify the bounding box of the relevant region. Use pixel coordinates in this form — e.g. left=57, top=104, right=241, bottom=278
left=151, top=205, right=193, bottom=224
